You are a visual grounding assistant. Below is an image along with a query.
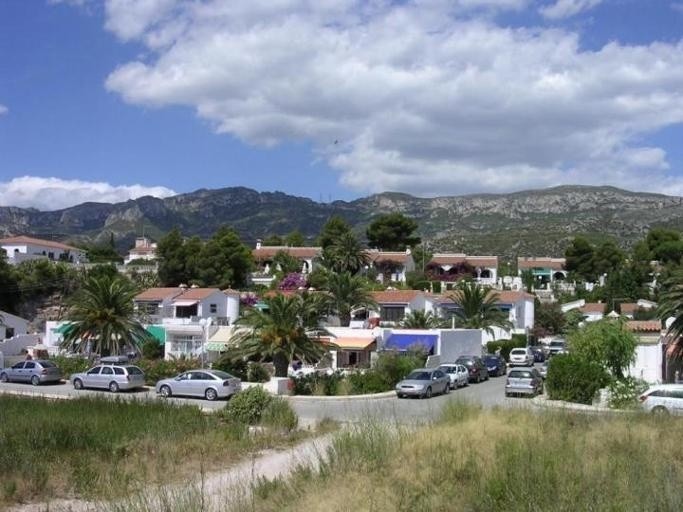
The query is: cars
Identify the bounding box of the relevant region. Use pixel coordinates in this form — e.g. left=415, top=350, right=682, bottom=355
left=156, top=369, right=241, bottom=400
left=70, top=365, right=145, bottom=392
left=637, top=384, right=683, bottom=417
left=0, top=360, right=59, bottom=385
left=395, top=339, right=567, bottom=399
